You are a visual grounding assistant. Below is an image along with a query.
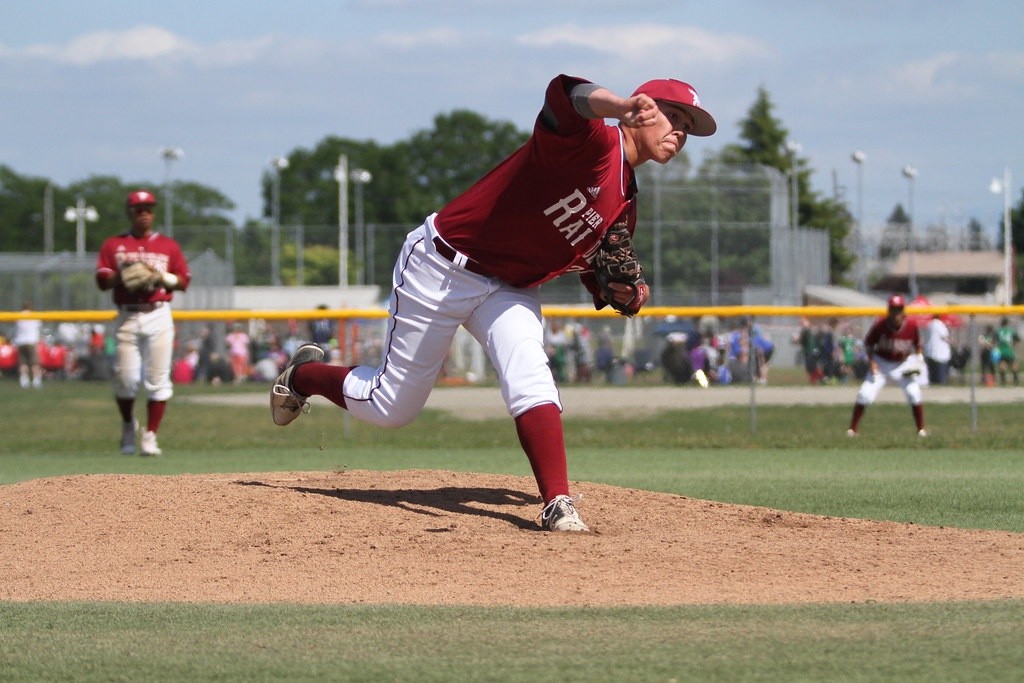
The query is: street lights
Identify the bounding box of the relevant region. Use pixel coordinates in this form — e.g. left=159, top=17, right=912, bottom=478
left=166, top=145, right=183, bottom=235
left=852, top=151, right=866, bottom=292
left=272, top=158, right=291, bottom=286
left=903, top=166, right=918, bottom=298
left=790, top=142, right=804, bottom=305
left=64, top=208, right=101, bottom=255
left=333, top=155, right=371, bottom=284
left=990, top=175, right=1012, bottom=308
left=45, top=167, right=76, bottom=256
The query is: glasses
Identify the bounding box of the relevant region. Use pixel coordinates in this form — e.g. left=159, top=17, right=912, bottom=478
left=133, top=206, right=153, bottom=214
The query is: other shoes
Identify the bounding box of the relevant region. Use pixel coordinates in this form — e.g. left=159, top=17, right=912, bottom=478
left=847, top=429, right=855, bottom=437
left=918, top=429, right=926, bottom=438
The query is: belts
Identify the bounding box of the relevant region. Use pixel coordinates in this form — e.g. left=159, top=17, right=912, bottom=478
left=431, top=237, right=521, bottom=289
left=119, top=301, right=162, bottom=311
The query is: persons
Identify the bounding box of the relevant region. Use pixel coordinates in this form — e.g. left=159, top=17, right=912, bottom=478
left=546, top=320, right=614, bottom=385
left=272, top=73, right=716, bottom=533
left=925, top=315, right=953, bottom=383
left=793, top=317, right=871, bottom=383
left=96, top=192, right=191, bottom=453
left=655, top=313, right=774, bottom=388
left=848, top=295, right=926, bottom=437
left=0, top=302, right=114, bottom=391
left=171, top=305, right=344, bottom=384
left=977, top=317, right=1020, bottom=384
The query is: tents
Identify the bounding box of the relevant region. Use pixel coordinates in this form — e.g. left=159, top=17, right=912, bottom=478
left=876, top=296, right=963, bottom=331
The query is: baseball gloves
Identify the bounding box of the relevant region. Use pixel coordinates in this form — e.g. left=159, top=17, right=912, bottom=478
left=119, top=262, right=154, bottom=294
left=577, top=222, right=646, bottom=316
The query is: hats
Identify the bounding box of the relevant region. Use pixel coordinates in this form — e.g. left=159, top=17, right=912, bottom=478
left=628, top=79, right=716, bottom=137
left=889, top=296, right=903, bottom=309
left=128, top=191, right=158, bottom=207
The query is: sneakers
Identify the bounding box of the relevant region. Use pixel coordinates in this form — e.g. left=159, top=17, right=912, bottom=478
left=119, top=418, right=139, bottom=454
left=536, top=494, right=590, bottom=534
left=269, top=343, right=325, bottom=426
left=140, top=427, right=161, bottom=456
